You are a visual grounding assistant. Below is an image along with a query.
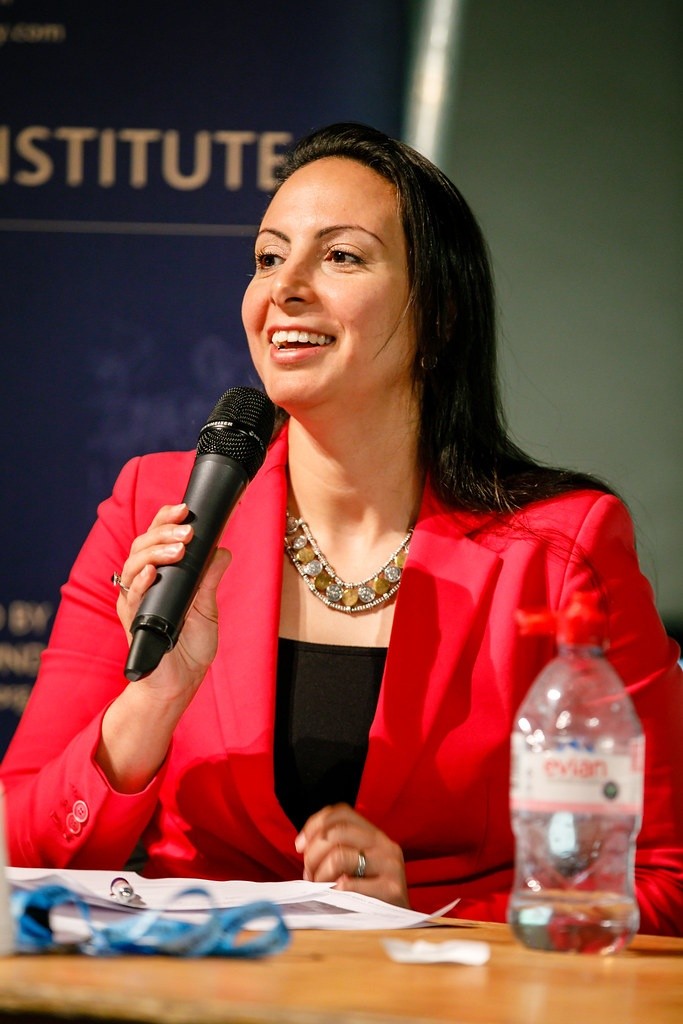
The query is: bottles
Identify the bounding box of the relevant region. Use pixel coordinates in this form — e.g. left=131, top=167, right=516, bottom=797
left=506, top=612, right=647, bottom=955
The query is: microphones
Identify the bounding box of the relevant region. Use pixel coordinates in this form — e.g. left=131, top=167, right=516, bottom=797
left=124, top=387, right=276, bottom=682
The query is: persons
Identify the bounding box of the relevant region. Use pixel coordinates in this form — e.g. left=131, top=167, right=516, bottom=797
left=1, top=121, right=683, bottom=937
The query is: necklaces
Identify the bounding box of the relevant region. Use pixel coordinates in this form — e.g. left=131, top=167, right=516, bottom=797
left=284, top=508, right=415, bottom=616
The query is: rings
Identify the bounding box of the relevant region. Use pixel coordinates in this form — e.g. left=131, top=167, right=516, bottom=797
left=352, top=847, right=366, bottom=878
left=110, top=571, right=129, bottom=591
